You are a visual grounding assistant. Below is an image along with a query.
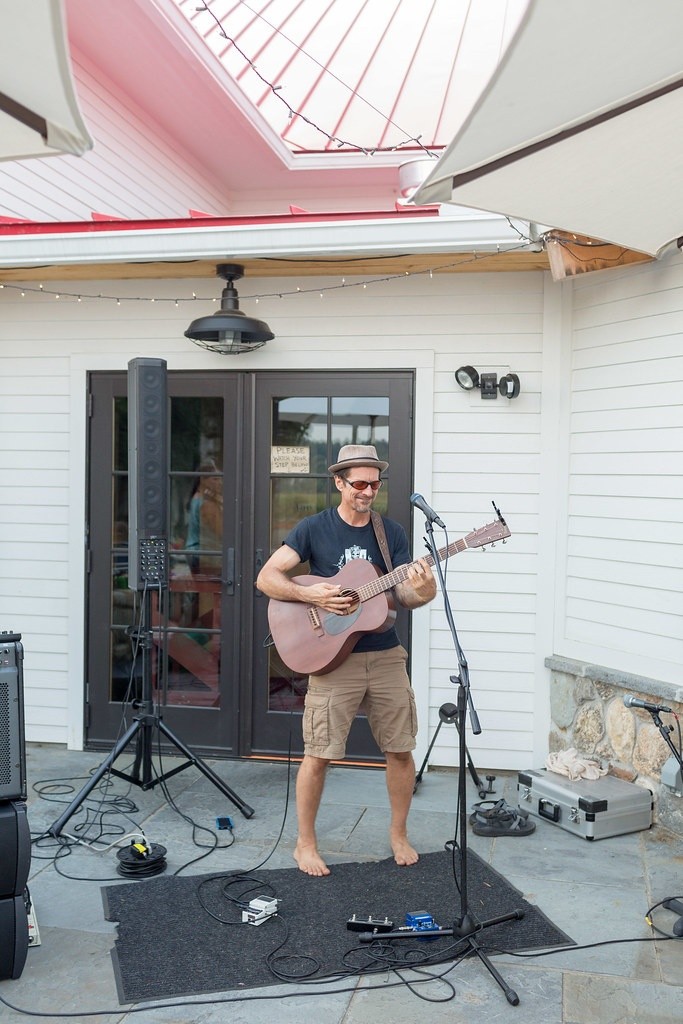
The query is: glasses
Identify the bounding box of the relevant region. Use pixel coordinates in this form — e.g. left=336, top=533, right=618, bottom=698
left=337, top=475, right=384, bottom=490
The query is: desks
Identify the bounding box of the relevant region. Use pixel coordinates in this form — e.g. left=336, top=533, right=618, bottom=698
left=148, top=567, right=305, bottom=713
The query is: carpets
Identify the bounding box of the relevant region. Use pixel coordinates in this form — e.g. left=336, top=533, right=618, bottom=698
left=99, top=846, right=577, bottom=1006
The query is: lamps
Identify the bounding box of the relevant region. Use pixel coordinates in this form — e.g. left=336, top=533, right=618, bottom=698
left=455, top=365, right=521, bottom=400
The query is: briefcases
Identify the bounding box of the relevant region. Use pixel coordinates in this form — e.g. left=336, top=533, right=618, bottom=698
left=516, top=769, right=654, bottom=841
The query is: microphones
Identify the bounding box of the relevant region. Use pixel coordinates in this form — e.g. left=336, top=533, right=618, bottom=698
left=411, top=493, right=447, bottom=529
left=622, top=694, right=672, bottom=712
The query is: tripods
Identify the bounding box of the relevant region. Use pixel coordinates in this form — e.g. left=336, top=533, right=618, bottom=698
left=48, top=540, right=256, bottom=837
left=359, top=522, right=525, bottom=1005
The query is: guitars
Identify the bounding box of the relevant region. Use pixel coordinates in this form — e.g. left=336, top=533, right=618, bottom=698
left=266, top=502, right=512, bottom=676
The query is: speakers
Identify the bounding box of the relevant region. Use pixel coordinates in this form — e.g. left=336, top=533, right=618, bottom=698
left=0, top=642, right=33, bottom=981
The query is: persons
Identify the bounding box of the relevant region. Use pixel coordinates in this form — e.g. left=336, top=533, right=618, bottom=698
left=257, top=445, right=436, bottom=877
left=183, top=459, right=225, bottom=587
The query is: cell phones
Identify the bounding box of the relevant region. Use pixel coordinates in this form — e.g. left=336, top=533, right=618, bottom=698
left=216, top=817, right=234, bottom=829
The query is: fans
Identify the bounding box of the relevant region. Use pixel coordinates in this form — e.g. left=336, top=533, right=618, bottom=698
left=183, top=263, right=275, bottom=355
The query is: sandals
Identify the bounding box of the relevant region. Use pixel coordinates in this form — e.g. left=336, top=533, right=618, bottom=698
left=469, top=798, right=529, bottom=825
left=472, top=811, right=536, bottom=838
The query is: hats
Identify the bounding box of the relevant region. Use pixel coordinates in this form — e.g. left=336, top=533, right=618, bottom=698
left=327, top=445, right=389, bottom=474
